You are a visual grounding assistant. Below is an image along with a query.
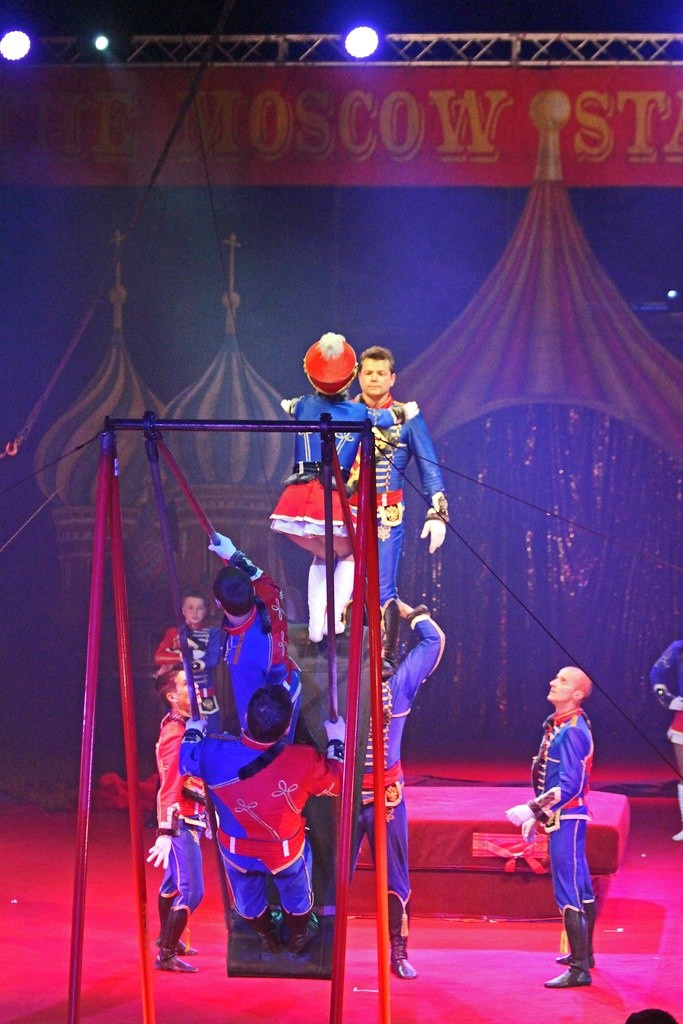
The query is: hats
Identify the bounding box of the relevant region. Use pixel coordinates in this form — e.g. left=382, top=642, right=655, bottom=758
left=303, top=333, right=359, bottom=394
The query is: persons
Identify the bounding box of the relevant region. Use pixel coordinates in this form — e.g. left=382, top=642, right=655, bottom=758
left=650, top=638, right=683, bottom=839
left=146, top=585, right=224, bottom=828
left=331, top=599, right=447, bottom=980
left=208, top=532, right=300, bottom=746
left=266, top=332, right=419, bottom=656
left=505, top=666, right=599, bottom=988
left=179, top=686, right=346, bottom=953
left=343, top=346, right=451, bottom=677
left=146, top=660, right=208, bottom=974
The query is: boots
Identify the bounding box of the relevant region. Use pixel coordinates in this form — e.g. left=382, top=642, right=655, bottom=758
left=323, top=560, right=355, bottom=635
left=234, top=901, right=283, bottom=953
left=379, top=598, right=401, bottom=682
left=307, top=564, right=326, bottom=642
left=154, top=889, right=198, bottom=955
left=154, top=905, right=199, bottom=973
left=280, top=893, right=322, bottom=953
left=671, top=784, right=683, bottom=842
left=544, top=904, right=592, bottom=988
left=386, top=889, right=419, bottom=979
left=556, top=896, right=595, bottom=967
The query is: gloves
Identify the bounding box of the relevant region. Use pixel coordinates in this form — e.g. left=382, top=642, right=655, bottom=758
left=185, top=715, right=208, bottom=732
left=208, top=532, right=237, bottom=560
left=505, top=804, right=535, bottom=826
left=192, top=658, right=206, bottom=673
left=324, top=716, right=346, bottom=744
left=420, top=519, right=447, bottom=553
left=280, top=399, right=291, bottom=413
left=204, top=812, right=219, bottom=839
left=403, top=401, right=419, bottom=420
left=146, top=835, right=172, bottom=869
left=521, top=817, right=538, bottom=844
left=192, top=650, right=206, bottom=661
left=668, top=696, right=683, bottom=711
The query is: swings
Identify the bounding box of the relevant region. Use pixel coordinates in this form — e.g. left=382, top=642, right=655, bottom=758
left=147, top=429, right=352, bottom=981
